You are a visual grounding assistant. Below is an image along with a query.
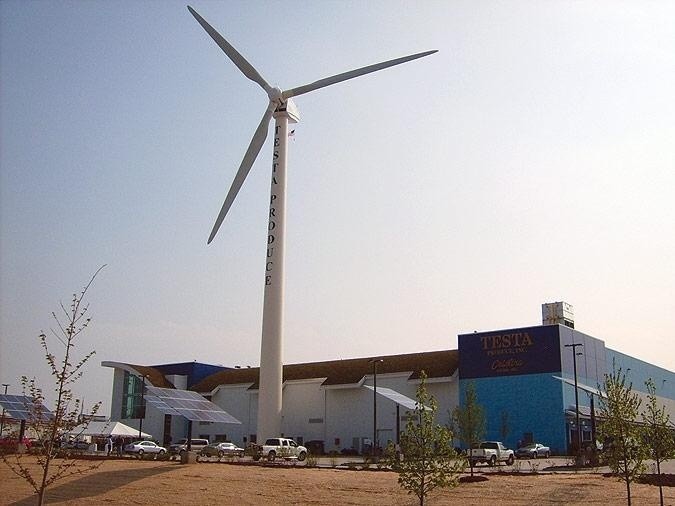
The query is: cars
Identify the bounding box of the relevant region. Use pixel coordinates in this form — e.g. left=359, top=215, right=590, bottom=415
left=125, top=440, right=168, bottom=455
left=0, top=432, right=92, bottom=448
left=515, top=443, right=551, bottom=459
left=201, top=442, right=246, bottom=458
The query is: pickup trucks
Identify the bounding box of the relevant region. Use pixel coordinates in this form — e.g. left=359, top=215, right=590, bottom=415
left=250, top=438, right=308, bottom=461
left=465, top=441, right=516, bottom=466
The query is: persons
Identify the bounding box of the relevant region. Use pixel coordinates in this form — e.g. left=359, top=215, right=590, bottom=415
left=106, top=434, right=114, bottom=457
left=115, top=434, right=125, bottom=458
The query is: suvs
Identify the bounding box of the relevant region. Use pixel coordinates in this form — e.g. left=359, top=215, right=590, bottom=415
left=580, top=439, right=602, bottom=458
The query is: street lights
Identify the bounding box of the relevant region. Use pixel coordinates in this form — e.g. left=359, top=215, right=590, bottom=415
left=1, top=383, right=10, bottom=395
left=137, top=373, right=152, bottom=439
left=564, top=342, right=583, bottom=466
left=372, top=358, right=384, bottom=459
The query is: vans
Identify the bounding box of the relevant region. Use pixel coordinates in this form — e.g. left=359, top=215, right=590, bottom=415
left=169, top=439, right=209, bottom=457
